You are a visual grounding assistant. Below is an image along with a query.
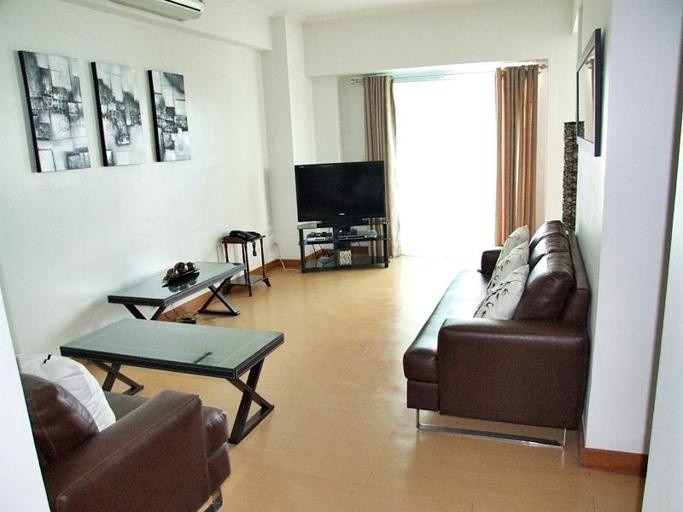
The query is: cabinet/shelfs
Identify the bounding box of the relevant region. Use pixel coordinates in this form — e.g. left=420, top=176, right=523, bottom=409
left=296, top=218, right=388, bottom=273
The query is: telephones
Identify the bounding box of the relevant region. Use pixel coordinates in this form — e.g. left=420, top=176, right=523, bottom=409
left=229, top=230, right=257, bottom=257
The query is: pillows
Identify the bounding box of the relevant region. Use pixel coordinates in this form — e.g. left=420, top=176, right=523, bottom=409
left=473, top=264, right=529, bottom=320
left=486, top=241, right=529, bottom=293
left=493, top=226, right=529, bottom=264
left=23, top=354, right=115, bottom=432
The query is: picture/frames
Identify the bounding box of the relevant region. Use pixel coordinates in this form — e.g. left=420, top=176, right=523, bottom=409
left=575, top=28, right=607, bottom=157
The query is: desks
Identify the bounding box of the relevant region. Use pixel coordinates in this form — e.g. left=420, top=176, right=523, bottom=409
left=221, top=236, right=271, bottom=296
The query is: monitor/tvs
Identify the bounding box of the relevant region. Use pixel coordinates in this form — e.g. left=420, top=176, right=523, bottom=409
left=294, top=160, right=386, bottom=228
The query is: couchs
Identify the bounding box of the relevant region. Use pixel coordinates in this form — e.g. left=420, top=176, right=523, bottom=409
left=402, top=221, right=590, bottom=449
left=19, top=371, right=231, bottom=511
